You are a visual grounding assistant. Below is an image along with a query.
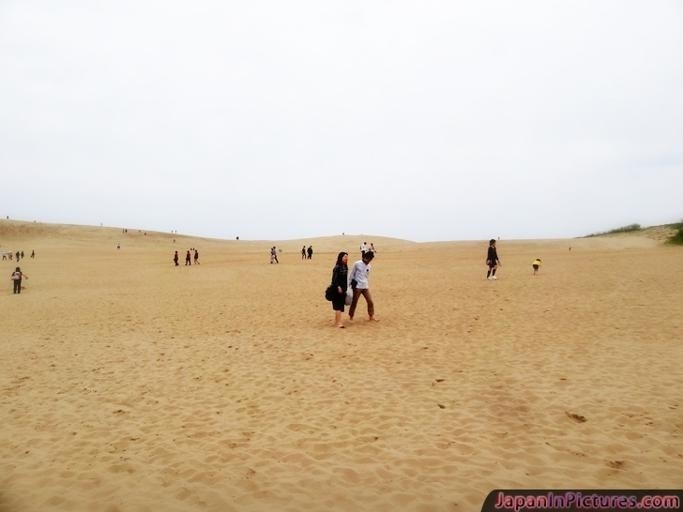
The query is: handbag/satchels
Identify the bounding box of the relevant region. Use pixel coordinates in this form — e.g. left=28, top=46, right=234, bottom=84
left=350, top=278, right=359, bottom=290
left=325, top=284, right=334, bottom=302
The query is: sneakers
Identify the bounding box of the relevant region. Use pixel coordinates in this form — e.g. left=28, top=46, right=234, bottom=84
left=487, top=276, right=496, bottom=281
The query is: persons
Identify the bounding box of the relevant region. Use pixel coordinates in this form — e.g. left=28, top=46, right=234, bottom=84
left=15, top=251, right=19, bottom=262
left=11, top=266, right=28, bottom=294
left=301, top=245, right=306, bottom=260
left=20, top=251, right=25, bottom=259
left=273, top=245, right=278, bottom=263
left=30, top=250, right=35, bottom=259
left=173, top=250, right=180, bottom=265
left=532, top=257, right=544, bottom=273
left=307, top=245, right=313, bottom=260
left=346, top=250, right=377, bottom=323
left=270, top=247, right=276, bottom=264
left=184, top=250, right=191, bottom=265
left=359, top=241, right=369, bottom=254
left=368, top=243, right=376, bottom=253
left=329, top=251, right=349, bottom=328
left=486, top=238, right=503, bottom=279
left=193, top=250, right=199, bottom=264
